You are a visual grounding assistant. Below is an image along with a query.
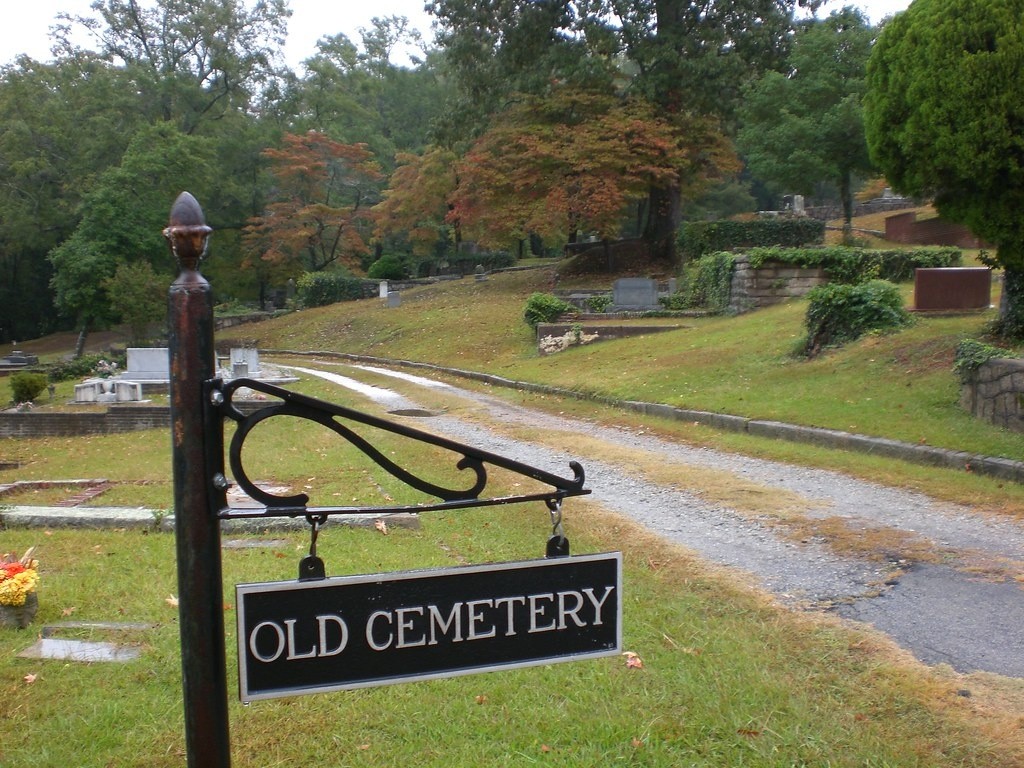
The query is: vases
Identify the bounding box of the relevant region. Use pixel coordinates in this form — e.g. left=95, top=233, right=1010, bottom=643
left=0, top=592, right=38, bottom=629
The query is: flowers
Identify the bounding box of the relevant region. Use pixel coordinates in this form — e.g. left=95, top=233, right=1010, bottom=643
left=0, top=546, right=40, bottom=607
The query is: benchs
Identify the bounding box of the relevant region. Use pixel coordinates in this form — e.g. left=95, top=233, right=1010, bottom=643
left=914, top=266, right=993, bottom=309
